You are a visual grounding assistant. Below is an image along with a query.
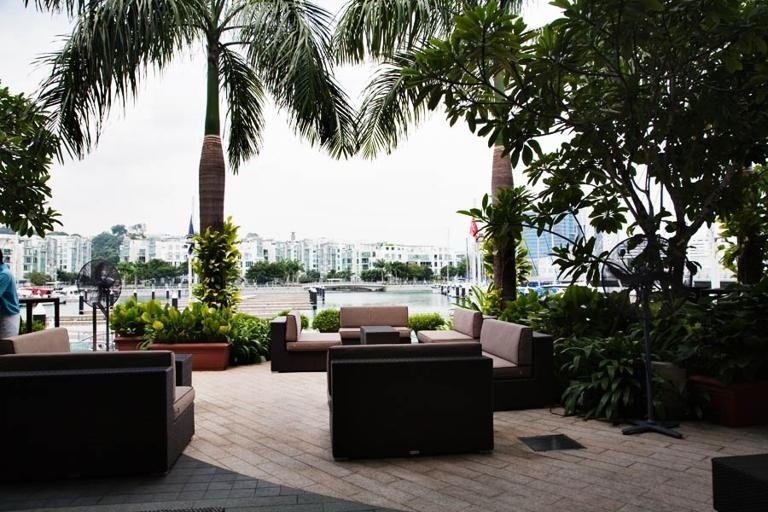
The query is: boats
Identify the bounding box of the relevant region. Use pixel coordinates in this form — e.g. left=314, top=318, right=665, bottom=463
left=16, top=285, right=114, bottom=306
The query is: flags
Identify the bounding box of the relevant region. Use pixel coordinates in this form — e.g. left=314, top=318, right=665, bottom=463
left=469, top=216, right=479, bottom=242
left=187, top=214, right=195, bottom=256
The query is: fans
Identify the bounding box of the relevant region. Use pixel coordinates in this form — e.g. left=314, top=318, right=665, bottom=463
left=77, top=260, right=122, bottom=351
left=601, top=234, right=692, bottom=439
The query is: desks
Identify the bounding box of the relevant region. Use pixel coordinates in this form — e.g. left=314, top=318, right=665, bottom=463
left=18, top=295, right=61, bottom=333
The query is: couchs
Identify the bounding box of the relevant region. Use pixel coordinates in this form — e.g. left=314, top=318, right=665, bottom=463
left=327, top=344, right=494, bottom=460
left=0, top=328, right=196, bottom=479
left=337, top=305, right=413, bottom=343
left=473, top=320, right=553, bottom=392
left=273, top=314, right=343, bottom=372
left=417, top=306, right=496, bottom=343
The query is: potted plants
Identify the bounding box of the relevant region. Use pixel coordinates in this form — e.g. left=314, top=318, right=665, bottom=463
left=109, top=295, right=243, bottom=372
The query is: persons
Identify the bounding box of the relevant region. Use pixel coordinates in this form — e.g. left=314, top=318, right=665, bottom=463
left=0, top=249, right=22, bottom=341
